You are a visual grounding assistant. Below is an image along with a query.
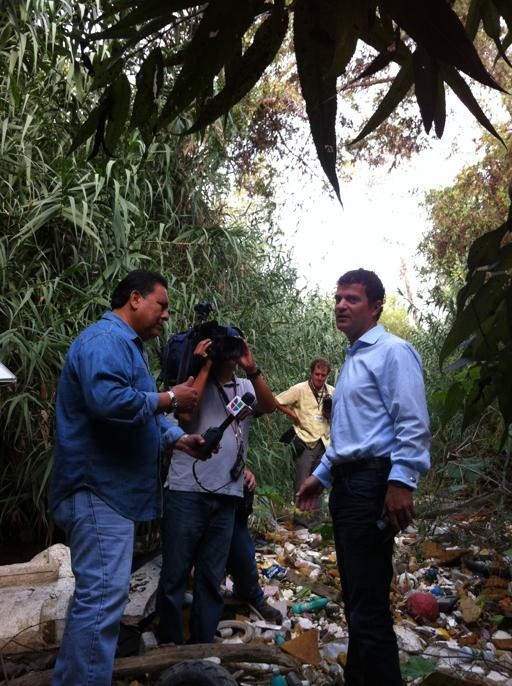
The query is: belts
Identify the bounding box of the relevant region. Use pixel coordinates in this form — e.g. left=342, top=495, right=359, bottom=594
left=330, top=457, right=391, bottom=475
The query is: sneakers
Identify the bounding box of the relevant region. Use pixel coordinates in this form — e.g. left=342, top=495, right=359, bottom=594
left=249, top=597, right=283, bottom=622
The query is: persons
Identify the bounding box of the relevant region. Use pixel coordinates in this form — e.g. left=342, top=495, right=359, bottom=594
left=275, top=358, right=335, bottom=508
left=48, top=270, right=205, bottom=686
left=141, top=326, right=283, bottom=646
left=294, top=268, right=432, bottom=686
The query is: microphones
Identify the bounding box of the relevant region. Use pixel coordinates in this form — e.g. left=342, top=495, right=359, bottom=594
left=196, top=392, right=256, bottom=453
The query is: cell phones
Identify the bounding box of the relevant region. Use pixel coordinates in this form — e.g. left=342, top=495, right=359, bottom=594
left=231, top=456, right=246, bottom=482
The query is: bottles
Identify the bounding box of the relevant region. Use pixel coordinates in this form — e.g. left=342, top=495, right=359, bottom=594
left=459, top=646, right=495, bottom=662
